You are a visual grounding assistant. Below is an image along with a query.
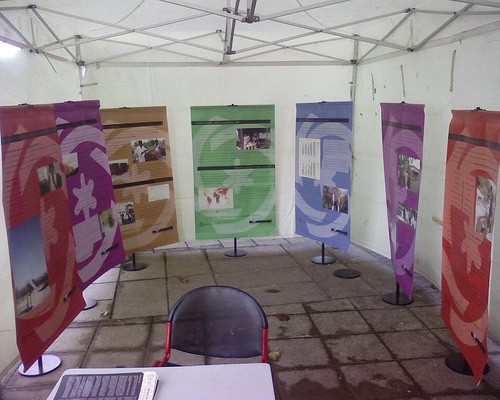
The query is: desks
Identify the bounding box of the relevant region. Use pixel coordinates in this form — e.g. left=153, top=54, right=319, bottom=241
left=46, top=362, right=275, bottom=400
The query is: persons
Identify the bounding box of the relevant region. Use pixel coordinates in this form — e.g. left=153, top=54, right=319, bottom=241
left=153, top=139, right=162, bottom=153
left=47, top=162, right=63, bottom=192
left=243, top=132, right=251, bottom=150
left=132, top=139, right=148, bottom=157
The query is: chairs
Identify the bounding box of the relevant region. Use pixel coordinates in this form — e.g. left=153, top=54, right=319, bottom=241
left=152, top=285, right=269, bottom=368
